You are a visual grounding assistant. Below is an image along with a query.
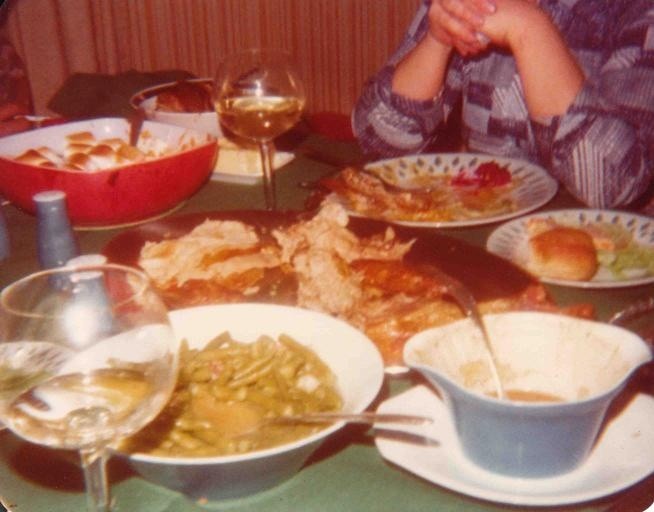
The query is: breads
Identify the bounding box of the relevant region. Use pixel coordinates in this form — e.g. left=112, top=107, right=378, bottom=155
left=525, top=225, right=598, bottom=282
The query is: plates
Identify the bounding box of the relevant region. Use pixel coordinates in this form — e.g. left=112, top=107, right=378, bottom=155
left=370, top=375, right=654, bottom=509
left=99, top=207, right=554, bottom=368
left=489, top=206, right=652, bottom=285
left=212, top=147, right=296, bottom=187
left=343, top=152, right=560, bottom=225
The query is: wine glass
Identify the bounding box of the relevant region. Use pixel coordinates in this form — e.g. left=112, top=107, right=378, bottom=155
left=210, top=47, right=307, bottom=207
left=0, top=261, right=180, bottom=512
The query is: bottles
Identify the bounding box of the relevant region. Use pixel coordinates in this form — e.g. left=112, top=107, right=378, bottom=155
left=31, top=187, right=115, bottom=340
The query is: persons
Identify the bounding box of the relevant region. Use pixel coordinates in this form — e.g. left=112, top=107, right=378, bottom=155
left=1, top=1, right=36, bottom=135
left=351, top=1, right=654, bottom=208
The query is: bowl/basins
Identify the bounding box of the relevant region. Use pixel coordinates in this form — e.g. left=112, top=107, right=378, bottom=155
left=56, top=302, right=385, bottom=503
left=0, top=114, right=220, bottom=230
left=398, top=309, right=654, bottom=478
left=128, top=79, right=278, bottom=142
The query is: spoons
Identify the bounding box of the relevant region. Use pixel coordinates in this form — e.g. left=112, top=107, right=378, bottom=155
left=231, top=405, right=432, bottom=440
